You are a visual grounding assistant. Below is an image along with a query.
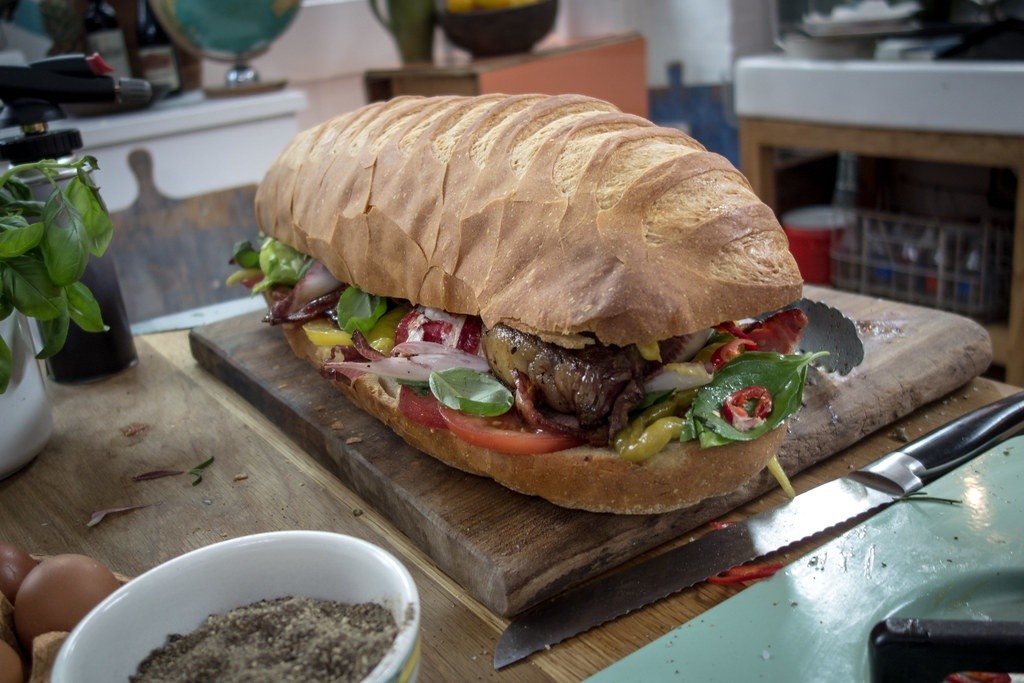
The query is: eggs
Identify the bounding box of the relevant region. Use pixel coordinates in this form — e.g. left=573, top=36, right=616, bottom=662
left=0, top=541, right=127, bottom=683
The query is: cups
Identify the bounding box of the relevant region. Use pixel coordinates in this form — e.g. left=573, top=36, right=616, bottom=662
left=50, top=531, right=421, bottom=683
left=783, top=210, right=848, bottom=284
left=369, top=0, right=434, bottom=64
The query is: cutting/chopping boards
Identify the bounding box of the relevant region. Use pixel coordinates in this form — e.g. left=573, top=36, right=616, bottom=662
left=189, top=283, right=991, bottom=626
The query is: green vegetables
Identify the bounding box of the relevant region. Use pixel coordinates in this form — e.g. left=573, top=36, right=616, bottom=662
left=0, top=154, right=127, bottom=396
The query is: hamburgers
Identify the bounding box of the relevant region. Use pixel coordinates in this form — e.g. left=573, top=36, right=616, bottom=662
left=225, top=94, right=834, bottom=516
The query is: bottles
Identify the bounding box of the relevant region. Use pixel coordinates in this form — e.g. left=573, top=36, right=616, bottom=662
left=85, top=0, right=132, bottom=89
left=135, top=0, right=181, bottom=97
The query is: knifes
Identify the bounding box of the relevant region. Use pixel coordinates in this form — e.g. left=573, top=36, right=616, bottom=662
left=494, top=388, right=1024, bottom=668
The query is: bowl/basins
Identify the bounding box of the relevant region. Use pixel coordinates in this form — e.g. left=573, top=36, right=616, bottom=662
left=433, top=1, right=559, bottom=57
